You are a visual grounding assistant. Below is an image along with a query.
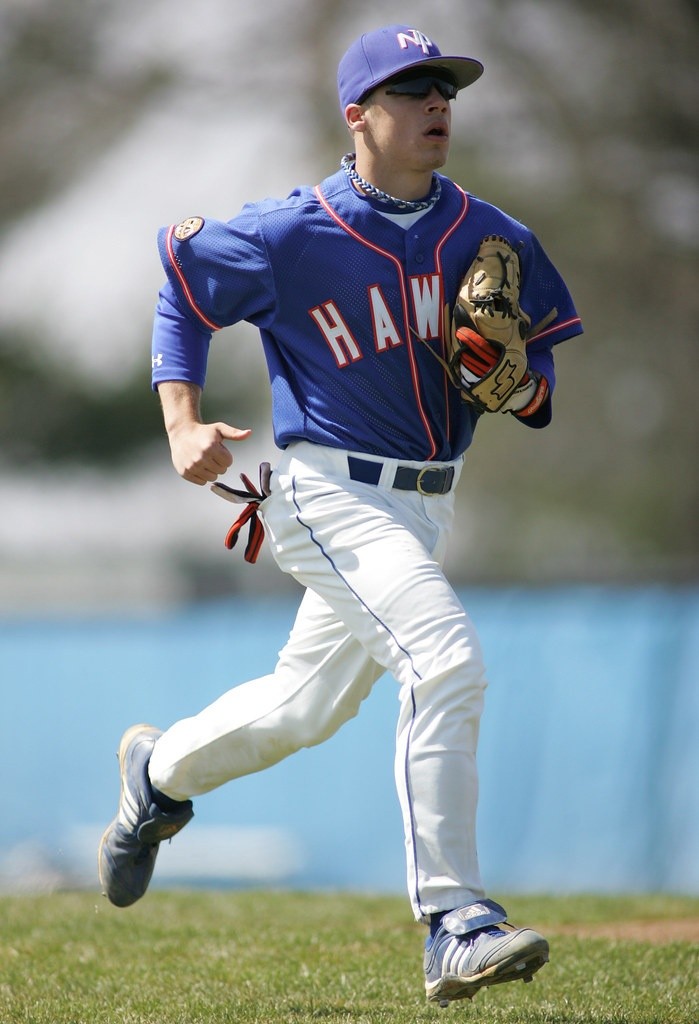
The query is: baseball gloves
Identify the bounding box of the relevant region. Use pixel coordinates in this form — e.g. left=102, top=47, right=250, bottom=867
left=439, top=224, right=548, bottom=417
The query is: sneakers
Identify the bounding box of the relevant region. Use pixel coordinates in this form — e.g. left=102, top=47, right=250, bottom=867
left=421, top=900, right=551, bottom=1008
left=98, top=723, right=195, bottom=908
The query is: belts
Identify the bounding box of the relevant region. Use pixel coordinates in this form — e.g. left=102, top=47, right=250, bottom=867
left=349, top=456, right=455, bottom=497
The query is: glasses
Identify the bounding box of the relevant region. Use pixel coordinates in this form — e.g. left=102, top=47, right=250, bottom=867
left=385, top=69, right=461, bottom=102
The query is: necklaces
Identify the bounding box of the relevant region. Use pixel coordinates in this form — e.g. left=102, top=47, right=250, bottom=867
left=341, top=151, right=442, bottom=211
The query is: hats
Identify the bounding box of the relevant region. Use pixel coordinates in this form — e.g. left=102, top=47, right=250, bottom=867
left=338, top=25, right=484, bottom=129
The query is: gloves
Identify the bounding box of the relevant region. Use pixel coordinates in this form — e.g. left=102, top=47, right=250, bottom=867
left=456, top=327, right=547, bottom=417
left=211, top=462, right=270, bottom=563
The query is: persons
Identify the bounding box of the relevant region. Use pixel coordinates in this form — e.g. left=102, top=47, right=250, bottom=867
left=93, top=26, right=584, bottom=1002
